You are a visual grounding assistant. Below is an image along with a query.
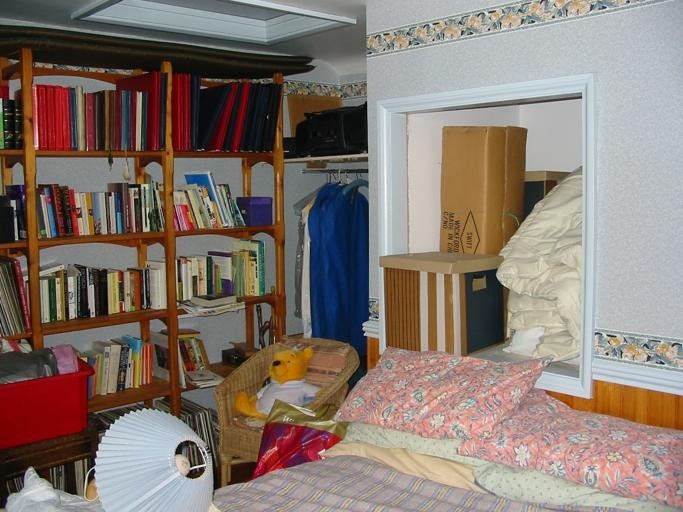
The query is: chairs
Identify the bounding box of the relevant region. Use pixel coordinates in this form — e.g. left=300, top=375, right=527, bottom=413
left=209, top=336, right=360, bottom=485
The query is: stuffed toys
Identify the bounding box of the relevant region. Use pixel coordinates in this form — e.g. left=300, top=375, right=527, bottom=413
left=235, top=343, right=322, bottom=423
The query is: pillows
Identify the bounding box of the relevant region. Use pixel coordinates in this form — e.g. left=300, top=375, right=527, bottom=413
left=476, top=462, right=661, bottom=509
left=458, top=386, right=683, bottom=505
left=292, top=344, right=350, bottom=390
left=330, top=347, right=554, bottom=439
left=344, top=420, right=491, bottom=467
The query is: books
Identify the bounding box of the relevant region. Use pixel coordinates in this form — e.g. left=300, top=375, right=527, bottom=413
left=3, top=69, right=284, bottom=153
left=1, top=327, right=220, bottom=512
left=1, top=238, right=266, bottom=335
left=2, top=172, right=246, bottom=240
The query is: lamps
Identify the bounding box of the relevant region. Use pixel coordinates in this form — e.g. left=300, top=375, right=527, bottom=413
left=84, top=408, right=215, bottom=511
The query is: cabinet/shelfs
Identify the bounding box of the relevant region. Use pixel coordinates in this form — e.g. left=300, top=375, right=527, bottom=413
left=0, top=25, right=313, bottom=424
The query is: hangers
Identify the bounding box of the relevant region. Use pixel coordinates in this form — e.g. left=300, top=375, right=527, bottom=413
left=289, top=168, right=368, bottom=215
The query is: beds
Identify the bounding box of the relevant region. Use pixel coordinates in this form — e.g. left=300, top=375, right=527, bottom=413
left=207, top=446, right=674, bottom=510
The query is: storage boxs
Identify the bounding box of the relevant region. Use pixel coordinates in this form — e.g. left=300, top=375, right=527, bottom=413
left=438, top=121, right=573, bottom=257
left=0, top=357, right=95, bottom=451
left=377, top=254, right=507, bottom=359
